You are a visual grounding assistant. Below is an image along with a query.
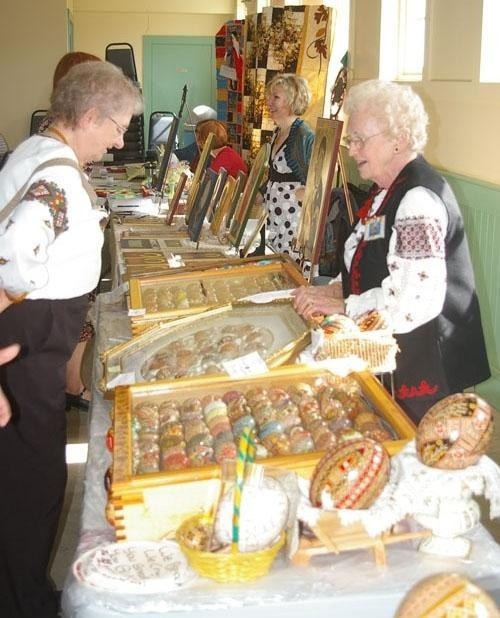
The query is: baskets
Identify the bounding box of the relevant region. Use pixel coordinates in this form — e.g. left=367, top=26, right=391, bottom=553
left=178, top=424, right=285, bottom=583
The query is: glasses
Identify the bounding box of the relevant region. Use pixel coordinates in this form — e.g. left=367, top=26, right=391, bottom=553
left=343, top=130, right=390, bottom=149
left=108, top=115, right=129, bottom=135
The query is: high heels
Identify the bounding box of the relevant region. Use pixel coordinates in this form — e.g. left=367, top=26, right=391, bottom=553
left=65, top=386, right=92, bottom=412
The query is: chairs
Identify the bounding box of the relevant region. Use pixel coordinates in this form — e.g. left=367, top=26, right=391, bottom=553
left=30, top=43, right=180, bottom=164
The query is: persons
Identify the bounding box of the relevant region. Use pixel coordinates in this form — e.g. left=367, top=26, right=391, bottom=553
left=39, top=51, right=108, bottom=411
left=0, top=60, right=145, bottom=618
left=289, top=78, right=493, bottom=429
left=197, top=121, right=265, bottom=206
left=254, top=73, right=317, bottom=282
left=0, top=343, right=20, bottom=428
left=173, top=118, right=217, bottom=174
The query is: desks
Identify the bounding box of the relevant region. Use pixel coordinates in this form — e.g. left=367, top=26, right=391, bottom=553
left=71, top=172, right=500, bottom=618
